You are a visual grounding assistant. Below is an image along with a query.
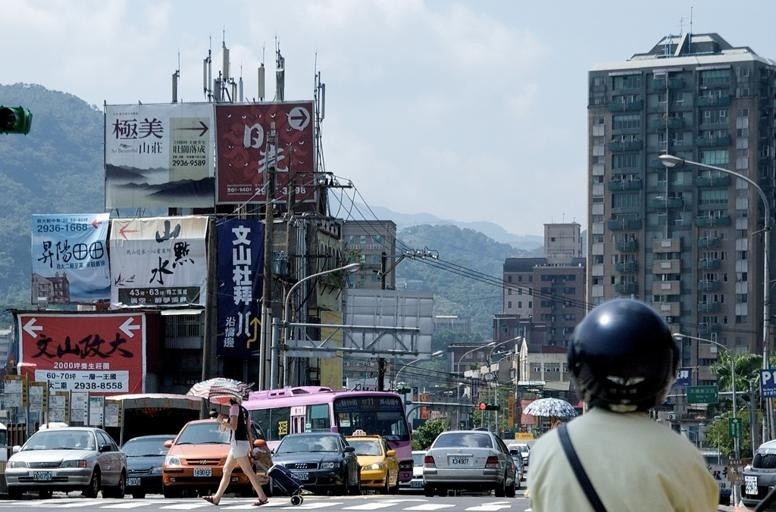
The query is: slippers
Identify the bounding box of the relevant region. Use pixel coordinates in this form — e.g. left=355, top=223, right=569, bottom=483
left=202, top=496, right=218, bottom=506
left=254, top=498, right=268, bottom=506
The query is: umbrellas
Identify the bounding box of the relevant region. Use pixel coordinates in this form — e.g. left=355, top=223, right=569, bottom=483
left=186, top=378, right=250, bottom=415
left=522, top=397, right=579, bottom=430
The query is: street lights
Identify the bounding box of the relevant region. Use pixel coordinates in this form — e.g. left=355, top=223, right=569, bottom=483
left=392, top=349, right=448, bottom=389
left=456, top=340, right=496, bottom=429
left=655, top=152, right=769, bottom=443
left=283, top=262, right=362, bottom=385
left=407, top=405, right=432, bottom=422
left=488, top=336, right=521, bottom=387
left=672, top=333, right=739, bottom=470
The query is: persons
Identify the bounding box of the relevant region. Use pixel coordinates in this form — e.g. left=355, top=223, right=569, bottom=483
left=203, top=398, right=269, bottom=507
left=526, top=298, right=720, bottom=512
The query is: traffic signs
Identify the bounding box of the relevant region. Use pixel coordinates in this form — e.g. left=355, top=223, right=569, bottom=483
left=729, top=417, right=743, bottom=439
left=687, top=386, right=719, bottom=404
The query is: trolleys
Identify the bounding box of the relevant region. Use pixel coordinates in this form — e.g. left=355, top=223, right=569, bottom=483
left=248, top=453, right=305, bottom=506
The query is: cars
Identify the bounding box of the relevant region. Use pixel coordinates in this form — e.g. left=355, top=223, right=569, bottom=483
left=120, top=434, right=180, bottom=499
left=4, top=427, right=127, bottom=500
left=399, top=431, right=529, bottom=497
left=343, top=429, right=398, bottom=495
left=162, top=418, right=273, bottom=498
left=271, top=431, right=361, bottom=497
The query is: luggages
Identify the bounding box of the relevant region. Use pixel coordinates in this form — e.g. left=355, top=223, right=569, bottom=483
left=252, top=456, right=303, bottom=505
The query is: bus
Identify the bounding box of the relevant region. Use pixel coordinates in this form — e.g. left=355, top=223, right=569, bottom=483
left=208, top=385, right=412, bottom=483
left=664, top=417, right=733, bottom=506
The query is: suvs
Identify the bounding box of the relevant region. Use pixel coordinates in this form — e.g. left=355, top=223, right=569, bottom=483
left=739, top=434, right=776, bottom=512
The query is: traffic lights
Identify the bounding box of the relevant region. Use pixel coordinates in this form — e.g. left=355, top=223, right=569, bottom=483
left=397, top=387, right=412, bottom=395
left=479, top=403, right=500, bottom=412
left=0, top=104, right=34, bottom=138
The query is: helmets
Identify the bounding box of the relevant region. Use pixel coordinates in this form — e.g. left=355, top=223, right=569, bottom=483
left=568, top=300, right=679, bottom=412
